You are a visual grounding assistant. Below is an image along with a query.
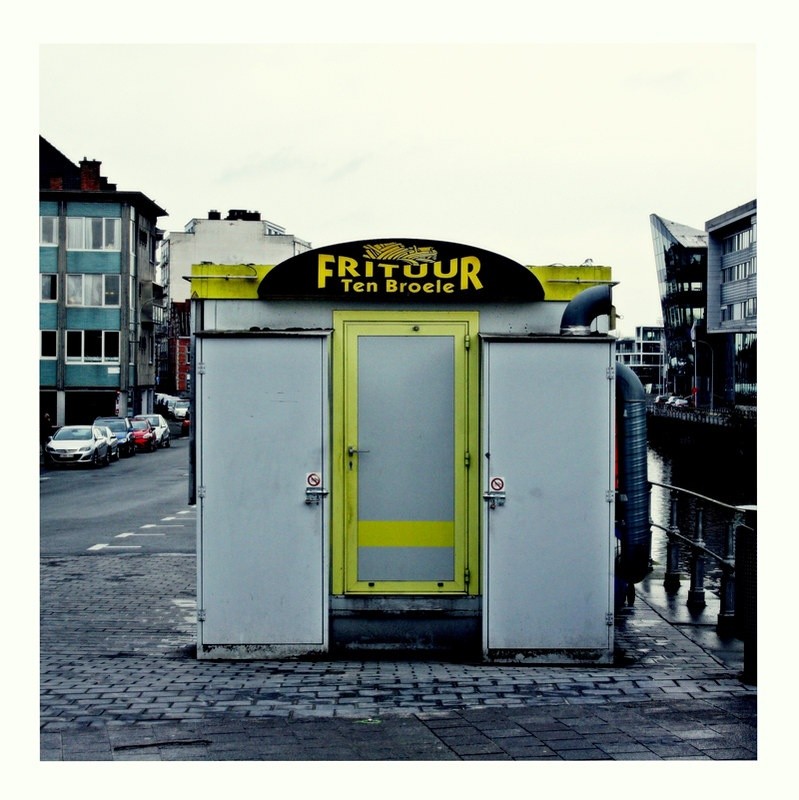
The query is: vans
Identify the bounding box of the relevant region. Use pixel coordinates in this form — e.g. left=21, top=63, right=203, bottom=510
left=93, top=416, right=136, bottom=458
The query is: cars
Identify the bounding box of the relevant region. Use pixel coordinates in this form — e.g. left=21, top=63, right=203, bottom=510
left=96, top=425, right=120, bottom=461
left=134, top=414, right=171, bottom=448
left=44, top=424, right=111, bottom=469
left=652, top=392, right=690, bottom=411
left=130, top=418, right=156, bottom=452
left=154, top=392, right=190, bottom=437
